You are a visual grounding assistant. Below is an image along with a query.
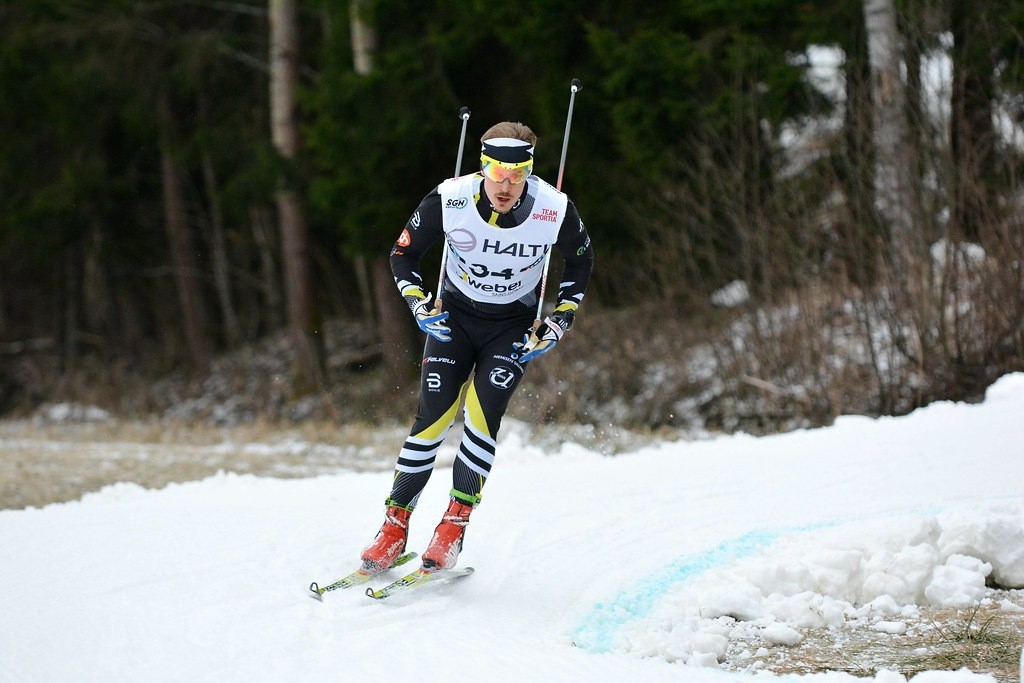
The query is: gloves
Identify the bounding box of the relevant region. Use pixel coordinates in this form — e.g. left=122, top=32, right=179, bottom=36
left=402, top=285, right=452, bottom=342
left=512, top=308, right=575, bottom=364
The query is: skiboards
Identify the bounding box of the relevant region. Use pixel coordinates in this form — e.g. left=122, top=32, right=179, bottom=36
left=308, top=551, right=475, bottom=602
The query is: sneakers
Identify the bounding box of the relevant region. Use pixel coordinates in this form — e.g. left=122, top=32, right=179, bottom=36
left=422, top=500, right=473, bottom=570
left=360, top=506, right=412, bottom=572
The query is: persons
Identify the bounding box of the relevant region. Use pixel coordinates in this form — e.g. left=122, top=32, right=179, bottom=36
left=359, top=121, right=595, bottom=575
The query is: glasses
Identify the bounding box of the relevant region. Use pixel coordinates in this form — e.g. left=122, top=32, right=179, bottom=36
left=480, top=155, right=534, bottom=185
